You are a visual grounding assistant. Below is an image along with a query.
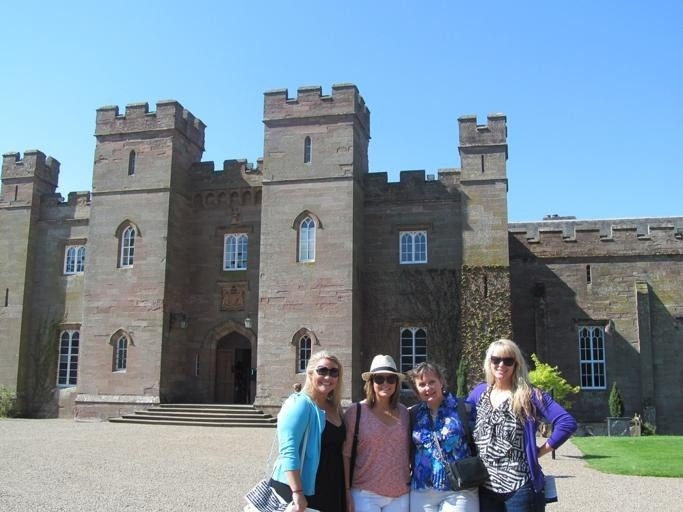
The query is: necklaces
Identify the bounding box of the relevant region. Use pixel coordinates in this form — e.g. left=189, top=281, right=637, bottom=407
left=375, top=400, right=392, bottom=410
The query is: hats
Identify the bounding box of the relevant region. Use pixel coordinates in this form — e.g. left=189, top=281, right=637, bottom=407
left=360, top=351, right=409, bottom=384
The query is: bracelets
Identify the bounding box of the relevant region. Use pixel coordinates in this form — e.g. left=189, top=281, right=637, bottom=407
left=290, top=489, right=302, bottom=494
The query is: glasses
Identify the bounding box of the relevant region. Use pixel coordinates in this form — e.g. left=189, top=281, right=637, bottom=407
left=311, top=363, right=341, bottom=380
left=488, top=355, right=517, bottom=367
left=368, top=373, right=401, bottom=385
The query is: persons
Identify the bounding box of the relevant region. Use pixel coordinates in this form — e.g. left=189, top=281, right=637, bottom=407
left=241, top=351, right=351, bottom=511
left=465, top=338, right=578, bottom=511
left=341, top=354, right=411, bottom=511
left=405, top=360, right=480, bottom=511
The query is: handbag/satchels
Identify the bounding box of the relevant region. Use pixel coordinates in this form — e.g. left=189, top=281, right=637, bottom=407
left=243, top=477, right=294, bottom=512
left=442, top=453, right=491, bottom=493
left=542, top=475, right=558, bottom=504
